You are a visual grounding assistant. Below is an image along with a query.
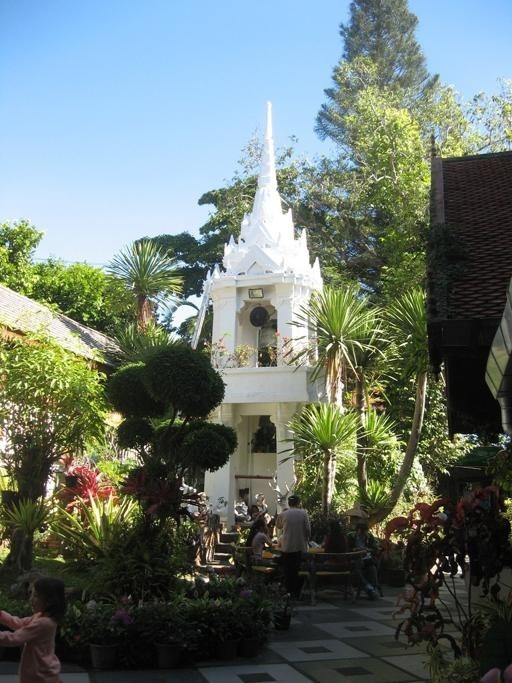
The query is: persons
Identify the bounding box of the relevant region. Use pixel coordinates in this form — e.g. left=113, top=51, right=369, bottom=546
left=272, top=494, right=310, bottom=596
left=346, top=522, right=380, bottom=601
left=252, top=507, right=277, bottom=539
left=0, top=578, right=72, bottom=683
left=321, top=520, right=349, bottom=552
left=247, top=519, right=273, bottom=565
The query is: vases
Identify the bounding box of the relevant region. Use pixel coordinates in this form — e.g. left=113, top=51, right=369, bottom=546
left=87, top=640, right=121, bottom=669
left=216, top=634, right=259, bottom=660
left=152, top=639, right=184, bottom=668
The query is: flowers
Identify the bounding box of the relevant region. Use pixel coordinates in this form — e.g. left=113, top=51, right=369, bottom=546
left=60, top=576, right=296, bottom=650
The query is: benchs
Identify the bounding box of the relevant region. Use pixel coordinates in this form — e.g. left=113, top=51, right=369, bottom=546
left=229, top=539, right=385, bottom=606
